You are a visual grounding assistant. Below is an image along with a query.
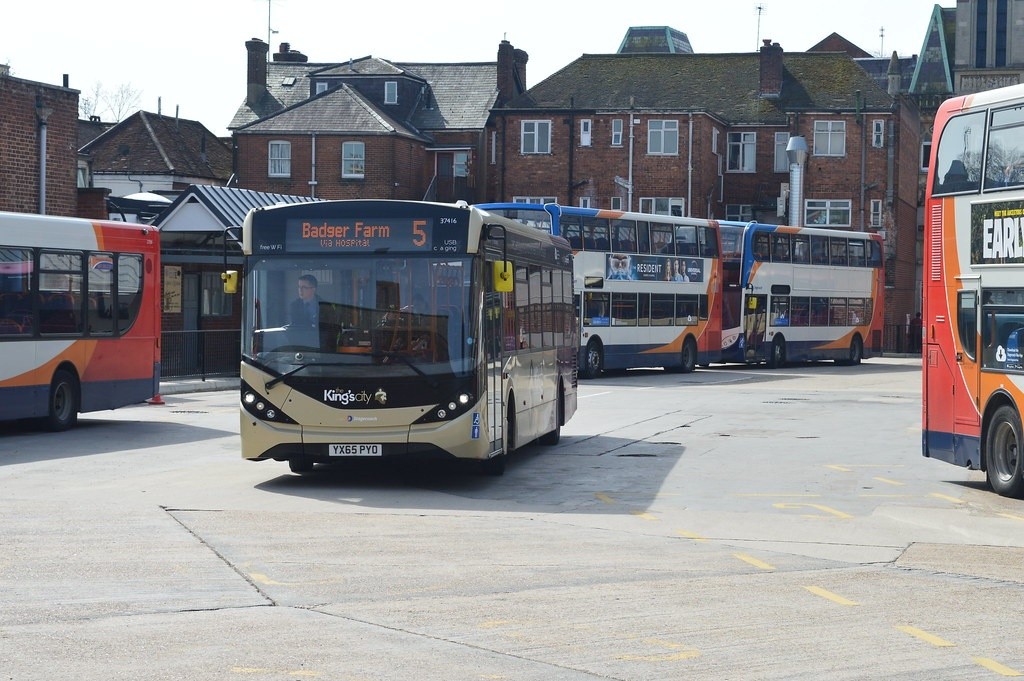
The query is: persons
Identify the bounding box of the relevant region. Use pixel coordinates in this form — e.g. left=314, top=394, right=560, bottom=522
left=908, top=312, right=923, bottom=353
left=519, top=318, right=526, bottom=350
left=378, top=288, right=507, bottom=365
left=658, top=258, right=692, bottom=282
left=288, top=274, right=341, bottom=354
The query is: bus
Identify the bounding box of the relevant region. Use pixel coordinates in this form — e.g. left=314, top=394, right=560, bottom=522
left=714, top=220, right=885, bottom=370
left=221, top=199, right=578, bottom=476
left=471, top=203, right=723, bottom=379
left=921, top=85, right=1024, bottom=498
left=0, top=211, right=161, bottom=432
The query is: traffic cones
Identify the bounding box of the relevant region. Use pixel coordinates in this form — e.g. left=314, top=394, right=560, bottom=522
left=148, top=393, right=165, bottom=405
